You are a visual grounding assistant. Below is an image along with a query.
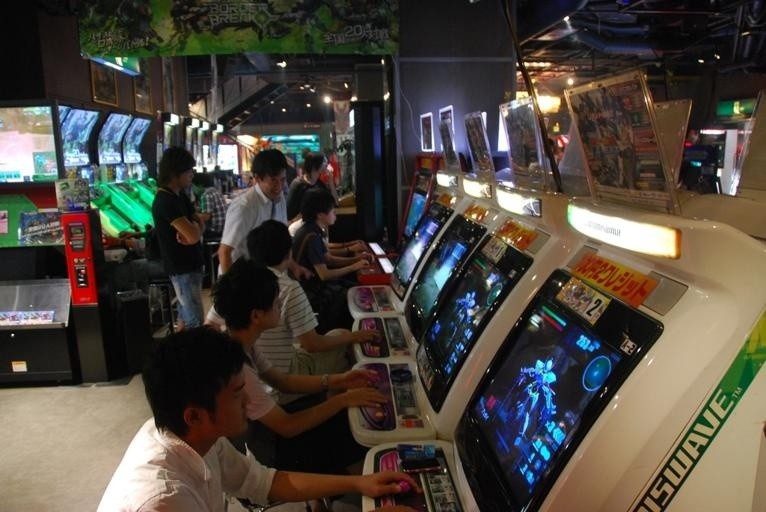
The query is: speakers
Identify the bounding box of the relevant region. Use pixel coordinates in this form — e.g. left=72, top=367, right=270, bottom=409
left=353, top=62, right=389, bottom=102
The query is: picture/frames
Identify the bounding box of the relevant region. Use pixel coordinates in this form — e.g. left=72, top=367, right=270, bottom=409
left=133, top=76, right=154, bottom=116
left=90, top=60, right=120, bottom=109
left=162, top=58, right=176, bottom=113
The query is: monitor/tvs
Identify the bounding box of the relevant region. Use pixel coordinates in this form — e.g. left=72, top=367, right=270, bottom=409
left=468, top=296, right=627, bottom=503
left=119, top=188, right=156, bottom=223
left=99, top=201, right=135, bottom=234
left=394, top=219, right=445, bottom=284
left=128, top=177, right=156, bottom=208
left=0, top=99, right=65, bottom=192
left=423, top=256, right=510, bottom=384
left=410, top=236, right=468, bottom=318
left=403, top=188, right=428, bottom=238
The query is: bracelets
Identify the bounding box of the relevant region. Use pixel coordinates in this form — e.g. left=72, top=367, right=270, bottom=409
left=321, top=372, right=330, bottom=391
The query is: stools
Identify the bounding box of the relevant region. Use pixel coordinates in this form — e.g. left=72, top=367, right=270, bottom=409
left=223, top=492, right=329, bottom=512
left=147, top=240, right=221, bottom=333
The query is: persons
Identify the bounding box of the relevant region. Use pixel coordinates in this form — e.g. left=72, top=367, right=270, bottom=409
left=202, top=217, right=378, bottom=405
left=144, top=148, right=215, bottom=328
left=534, top=354, right=558, bottom=386
left=207, top=256, right=390, bottom=501
left=454, top=289, right=481, bottom=317
left=677, top=127, right=715, bottom=191
left=572, top=87, right=638, bottom=192
left=191, top=140, right=377, bottom=282
left=98, top=327, right=424, bottom=511
